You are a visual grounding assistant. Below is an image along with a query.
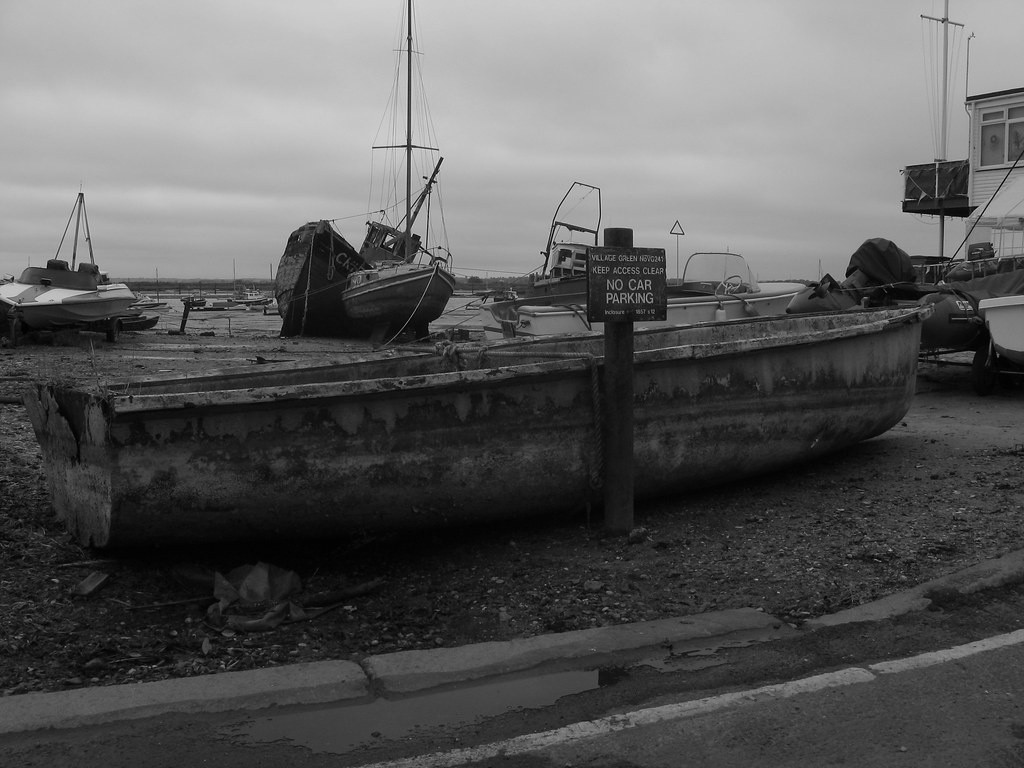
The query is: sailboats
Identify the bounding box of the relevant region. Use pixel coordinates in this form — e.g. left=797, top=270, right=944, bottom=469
left=273, top=0, right=455, bottom=339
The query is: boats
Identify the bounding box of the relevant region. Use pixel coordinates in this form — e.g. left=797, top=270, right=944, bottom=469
left=465, top=181, right=1024, bottom=367
left=0, top=188, right=147, bottom=328
left=128, top=257, right=275, bottom=310
left=18, top=299, right=936, bottom=555
